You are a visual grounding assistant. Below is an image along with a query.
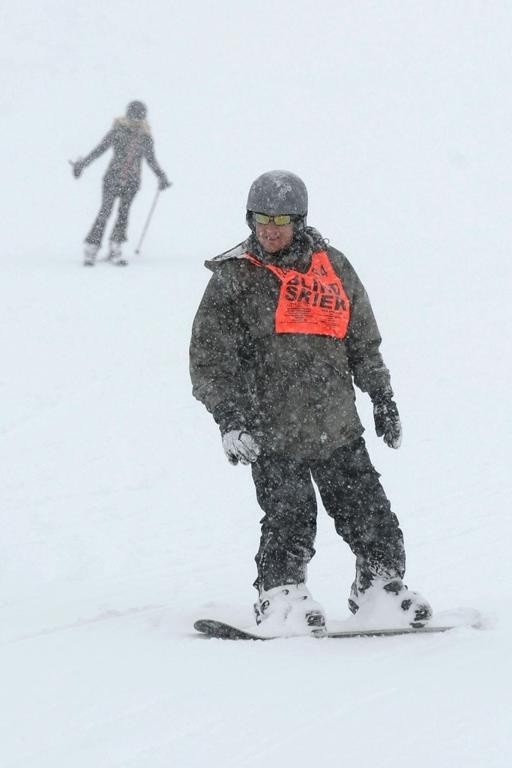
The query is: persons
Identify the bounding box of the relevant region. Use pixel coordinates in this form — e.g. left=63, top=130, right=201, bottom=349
left=71, top=100, right=170, bottom=263
left=190, top=168, right=432, bottom=630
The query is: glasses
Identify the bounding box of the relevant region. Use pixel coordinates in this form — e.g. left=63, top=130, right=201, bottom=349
left=248, top=212, right=306, bottom=225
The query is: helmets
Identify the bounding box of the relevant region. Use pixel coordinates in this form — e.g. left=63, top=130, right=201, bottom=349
left=246, top=171, right=308, bottom=230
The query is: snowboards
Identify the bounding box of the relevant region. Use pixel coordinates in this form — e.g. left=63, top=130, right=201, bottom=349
left=196, top=621, right=456, bottom=641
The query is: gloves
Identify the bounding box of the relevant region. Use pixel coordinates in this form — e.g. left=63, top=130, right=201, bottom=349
left=219, top=412, right=262, bottom=464
left=371, top=389, right=402, bottom=448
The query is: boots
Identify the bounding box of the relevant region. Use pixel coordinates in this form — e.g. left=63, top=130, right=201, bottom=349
left=82, top=241, right=97, bottom=265
left=253, top=582, right=325, bottom=631
left=348, top=580, right=433, bottom=627
left=110, top=240, right=127, bottom=265
